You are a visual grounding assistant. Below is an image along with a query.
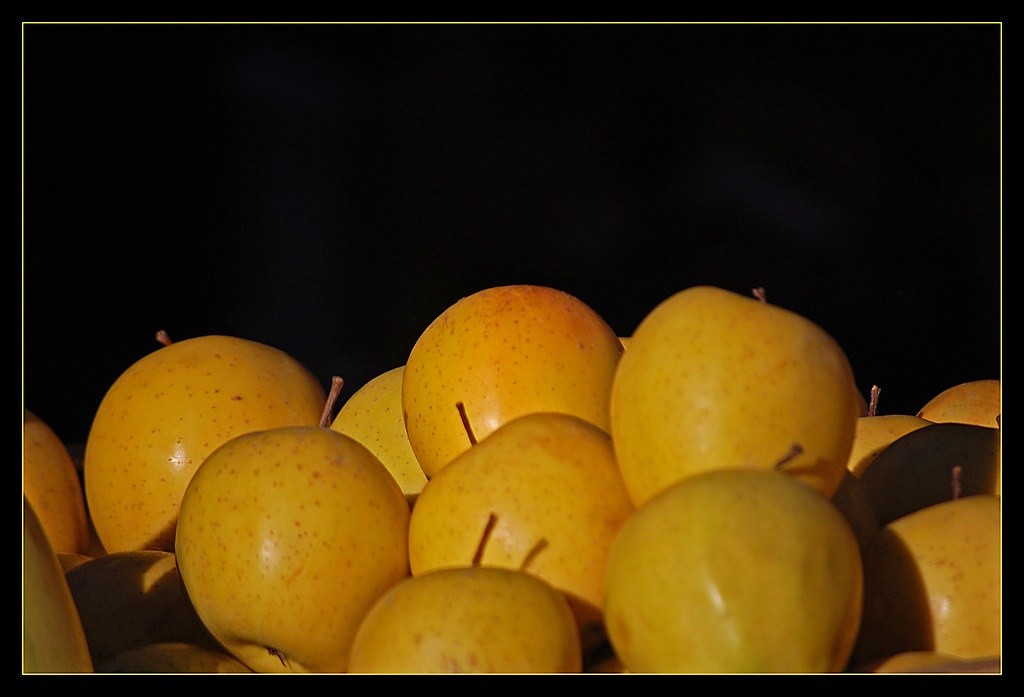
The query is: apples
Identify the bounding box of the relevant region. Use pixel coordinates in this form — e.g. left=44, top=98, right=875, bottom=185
left=22, top=282, right=1002, bottom=673
